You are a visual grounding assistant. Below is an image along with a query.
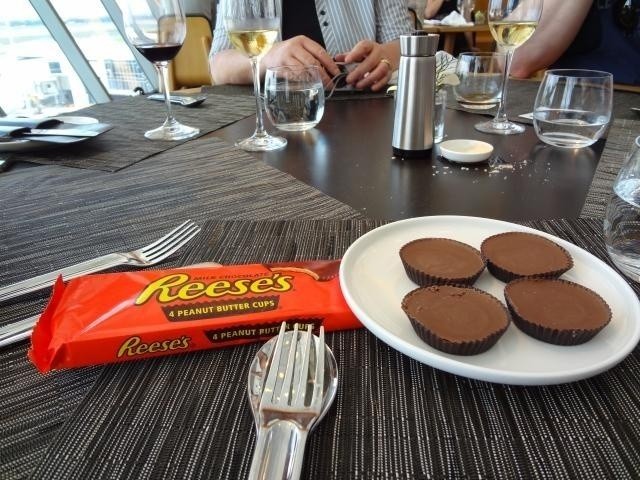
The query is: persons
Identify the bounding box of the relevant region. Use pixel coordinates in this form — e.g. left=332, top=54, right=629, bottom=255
left=208, top=0, right=425, bottom=95
left=493, top=0, right=639, bottom=80
left=424, top=0, right=477, bottom=58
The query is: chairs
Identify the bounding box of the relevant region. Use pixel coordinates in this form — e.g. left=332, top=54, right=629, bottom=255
left=155, top=12, right=217, bottom=91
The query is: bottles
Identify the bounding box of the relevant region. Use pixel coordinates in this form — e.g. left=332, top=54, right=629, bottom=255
left=467, top=3, right=485, bottom=25
left=390, top=31, right=439, bottom=161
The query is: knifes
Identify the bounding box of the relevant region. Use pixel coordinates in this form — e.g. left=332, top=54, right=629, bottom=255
left=20, top=126, right=100, bottom=137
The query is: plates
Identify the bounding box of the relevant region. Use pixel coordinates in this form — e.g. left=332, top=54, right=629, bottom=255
left=0, top=116, right=99, bottom=153
left=337, top=216, right=640, bottom=387
left=438, top=137, right=494, bottom=165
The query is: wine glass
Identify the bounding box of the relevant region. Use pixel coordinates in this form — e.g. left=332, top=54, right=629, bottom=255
left=222, top=0, right=288, bottom=153
left=124, top=0, right=199, bottom=142
left=473, top=0, right=545, bottom=135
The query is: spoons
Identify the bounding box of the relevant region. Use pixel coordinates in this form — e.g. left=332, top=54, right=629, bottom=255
left=1, top=261, right=223, bottom=348
left=147, top=92, right=207, bottom=106
left=248, top=330, right=339, bottom=478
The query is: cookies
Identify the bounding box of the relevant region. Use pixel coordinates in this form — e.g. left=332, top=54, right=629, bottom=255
left=400, top=232, right=611, bottom=356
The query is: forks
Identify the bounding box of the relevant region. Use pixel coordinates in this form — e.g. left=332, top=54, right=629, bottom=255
left=2, top=214, right=203, bottom=305
left=248, top=321, right=325, bottom=480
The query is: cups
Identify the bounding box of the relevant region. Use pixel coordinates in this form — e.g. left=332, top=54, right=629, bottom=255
left=533, top=68, right=613, bottom=149
left=455, top=52, right=507, bottom=110
left=264, top=64, right=326, bottom=132
left=264, top=121, right=328, bottom=198
left=603, top=136, right=640, bottom=287
left=434, top=88, right=446, bottom=143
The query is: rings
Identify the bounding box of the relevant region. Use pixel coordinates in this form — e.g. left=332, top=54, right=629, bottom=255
left=380, top=58, right=391, bottom=69
